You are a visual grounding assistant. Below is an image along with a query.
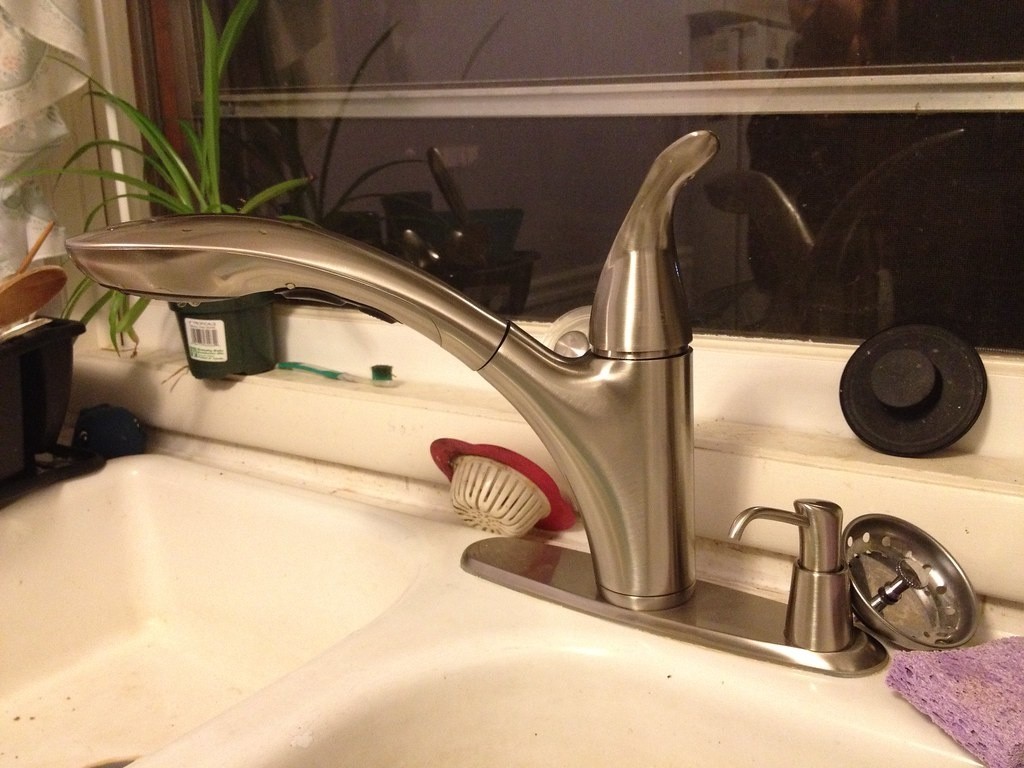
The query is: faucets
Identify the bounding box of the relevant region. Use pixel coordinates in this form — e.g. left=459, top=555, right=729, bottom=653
left=62, top=126, right=733, bottom=631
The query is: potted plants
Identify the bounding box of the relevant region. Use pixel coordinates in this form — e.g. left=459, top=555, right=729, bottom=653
left=0, top=1, right=325, bottom=378
left=223, top=11, right=455, bottom=264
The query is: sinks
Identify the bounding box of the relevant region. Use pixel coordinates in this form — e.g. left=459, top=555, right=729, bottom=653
left=238, top=542, right=975, bottom=767
left=0, top=435, right=448, bottom=768
